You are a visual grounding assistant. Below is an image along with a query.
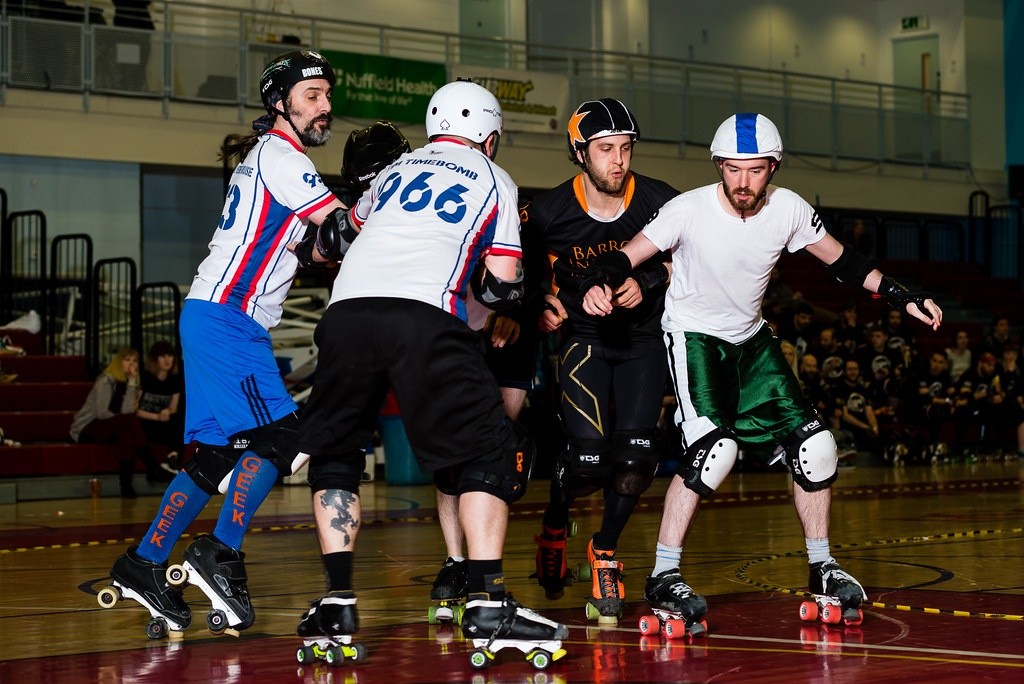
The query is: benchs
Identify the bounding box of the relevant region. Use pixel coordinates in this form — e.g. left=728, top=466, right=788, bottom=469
left=0, top=316, right=197, bottom=478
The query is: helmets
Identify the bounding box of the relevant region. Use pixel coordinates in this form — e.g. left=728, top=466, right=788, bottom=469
left=566, top=97, right=641, bottom=166
left=341, top=120, right=411, bottom=199
left=261, top=49, right=337, bottom=116
left=708, top=114, right=783, bottom=164
left=426, top=80, right=504, bottom=144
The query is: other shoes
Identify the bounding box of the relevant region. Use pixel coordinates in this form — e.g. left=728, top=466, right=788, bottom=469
left=161, top=456, right=183, bottom=475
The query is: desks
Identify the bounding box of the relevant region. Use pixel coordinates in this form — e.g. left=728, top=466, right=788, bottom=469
left=249, top=40, right=309, bottom=62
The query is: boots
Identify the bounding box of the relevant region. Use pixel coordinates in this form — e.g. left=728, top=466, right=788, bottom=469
left=116, top=457, right=138, bottom=498
left=140, top=450, right=174, bottom=486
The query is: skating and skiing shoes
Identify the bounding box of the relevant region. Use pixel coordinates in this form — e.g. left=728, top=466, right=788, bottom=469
left=799, top=558, right=870, bottom=626
left=120, top=641, right=190, bottom=684
left=97, top=544, right=193, bottom=638
left=639, top=635, right=709, bottom=684
left=470, top=669, right=570, bottom=684
left=428, top=621, right=467, bottom=656
left=799, top=626, right=869, bottom=684
left=296, top=665, right=359, bottom=684
left=166, top=533, right=255, bottom=637
left=429, top=558, right=468, bottom=622
left=577, top=537, right=627, bottom=626
left=294, top=591, right=366, bottom=663
left=530, top=519, right=579, bottom=599
left=460, top=589, right=570, bottom=668
left=637, top=569, right=709, bottom=637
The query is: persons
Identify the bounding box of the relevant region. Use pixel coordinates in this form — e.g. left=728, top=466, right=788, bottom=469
left=521, top=98, right=682, bottom=623
left=70, top=340, right=183, bottom=500
left=762, top=267, right=1024, bottom=466
left=96, top=51, right=347, bottom=641
left=287, top=78, right=570, bottom=666
left=844, top=220, right=864, bottom=248
left=111, top=0, right=155, bottom=91
left=581, top=113, right=943, bottom=639
